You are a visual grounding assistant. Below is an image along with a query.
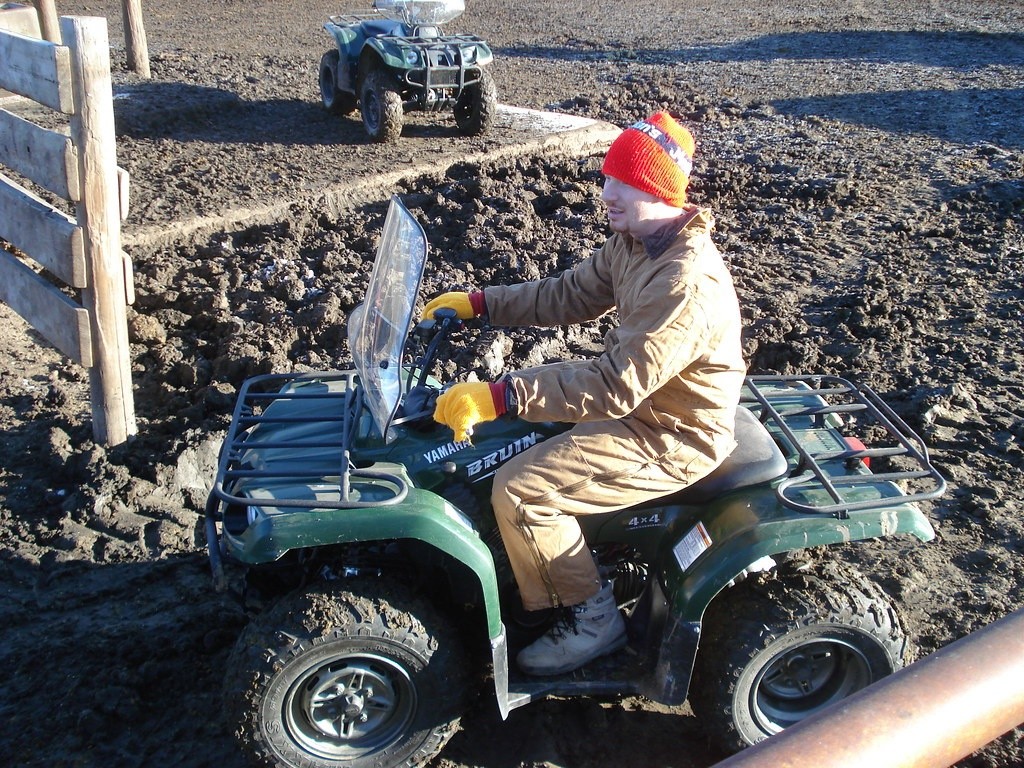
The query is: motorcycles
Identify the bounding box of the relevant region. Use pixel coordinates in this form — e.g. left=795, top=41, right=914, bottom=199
left=319, top=0, right=497, bottom=143
left=206, top=193, right=939, bottom=768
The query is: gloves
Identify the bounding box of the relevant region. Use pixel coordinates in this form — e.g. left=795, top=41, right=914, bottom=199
left=434, top=381, right=508, bottom=443
left=421, top=291, right=486, bottom=320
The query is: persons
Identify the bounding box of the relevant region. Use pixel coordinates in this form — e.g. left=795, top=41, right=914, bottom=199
left=421, top=112, right=746, bottom=675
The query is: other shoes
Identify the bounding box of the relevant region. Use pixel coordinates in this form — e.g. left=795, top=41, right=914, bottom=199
left=517, top=583, right=627, bottom=676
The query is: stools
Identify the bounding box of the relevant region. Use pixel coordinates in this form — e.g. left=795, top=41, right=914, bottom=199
left=360, top=18, right=397, bottom=37
left=679, top=401, right=791, bottom=507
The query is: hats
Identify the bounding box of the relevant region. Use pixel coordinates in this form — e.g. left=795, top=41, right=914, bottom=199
left=602, top=113, right=695, bottom=208
